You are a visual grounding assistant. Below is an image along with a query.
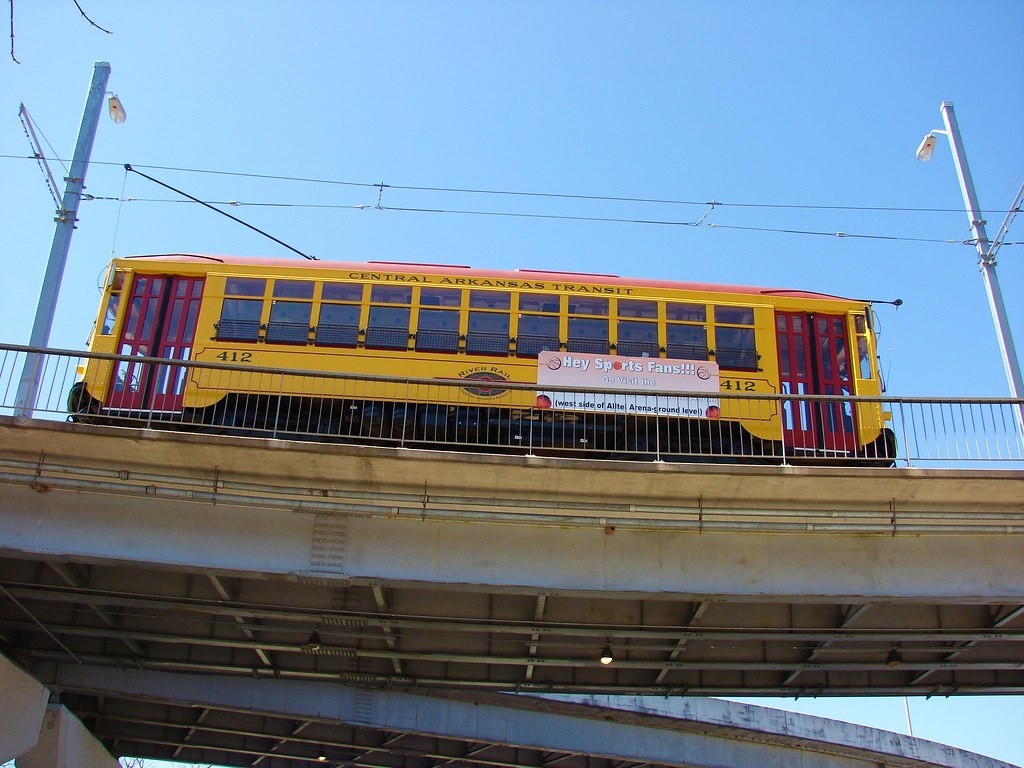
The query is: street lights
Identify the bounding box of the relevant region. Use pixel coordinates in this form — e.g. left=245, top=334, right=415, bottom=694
left=12, top=61, right=129, bottom=418
left=916, top=125, right=1023, bottom=436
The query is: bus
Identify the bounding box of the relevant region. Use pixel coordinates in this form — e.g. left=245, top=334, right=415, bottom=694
left=66, top=251, right=903, bottom=466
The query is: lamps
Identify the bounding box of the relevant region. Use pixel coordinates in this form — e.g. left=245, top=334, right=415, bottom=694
left=308, top=622, right=321, bottom=652
left=600, top=637, right=613, bottom=665
left=317, top=751, right=328, bottom=761
left=885, top=649, right=902, bottom=668
